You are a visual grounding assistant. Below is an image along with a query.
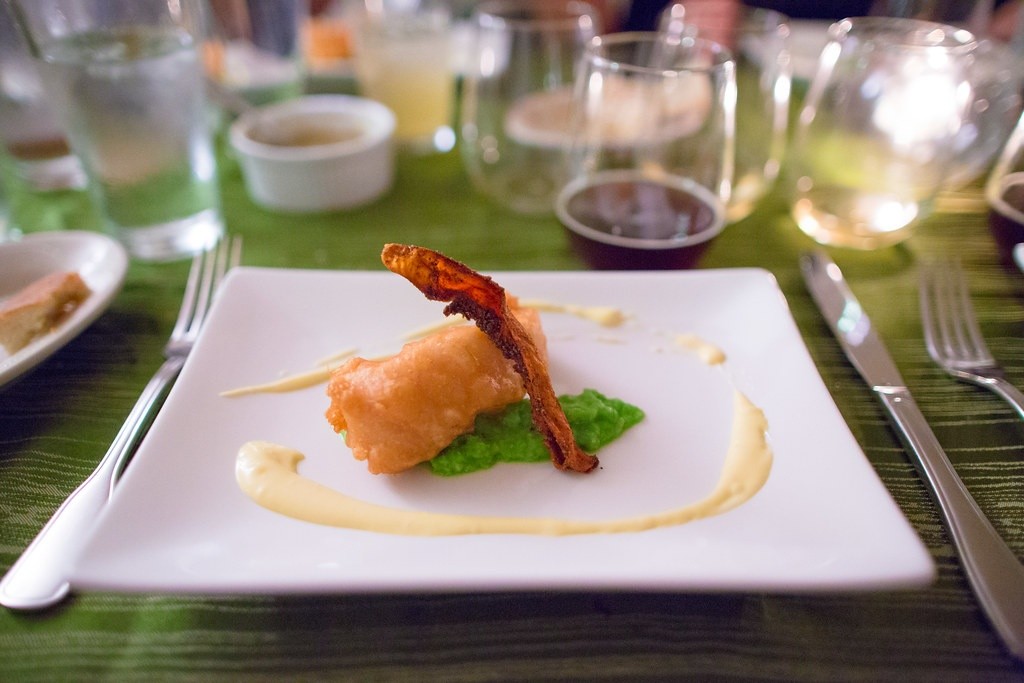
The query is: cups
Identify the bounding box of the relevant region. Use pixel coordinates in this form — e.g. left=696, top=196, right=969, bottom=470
left=341, top=0, right=462, bottom=160
left=652, top=2, right=790, bottom=229
left=551, top=31, right=737, bottom=270
left=1, top=26, right=90, bottom=194
left=982, top=107, right=1023, bottom=288
left=206, top=0, right=311, bottom=116
left=459, top=2, right=603, bottom=220
left=787, top=16, right=979, bottom=251
left=3, top=0, right=228, bottom=268
left=928, top=43, right=1021, bottom=217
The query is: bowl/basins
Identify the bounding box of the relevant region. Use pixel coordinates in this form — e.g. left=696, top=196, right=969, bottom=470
left=228, top=89, right=399, bottom=215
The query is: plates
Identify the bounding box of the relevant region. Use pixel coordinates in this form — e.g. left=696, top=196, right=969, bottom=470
left=62, top=263, right=939, bottom=595
left=1, top=230, right=132, bottom=390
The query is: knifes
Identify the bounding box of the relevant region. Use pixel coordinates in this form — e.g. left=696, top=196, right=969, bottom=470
left=797, top=240, right=1023, bottom=668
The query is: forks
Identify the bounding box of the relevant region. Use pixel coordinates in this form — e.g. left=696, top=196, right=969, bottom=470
left=908, top=250, right=1022, bottom=425
left=1, top=232, right=242, bottom=610
